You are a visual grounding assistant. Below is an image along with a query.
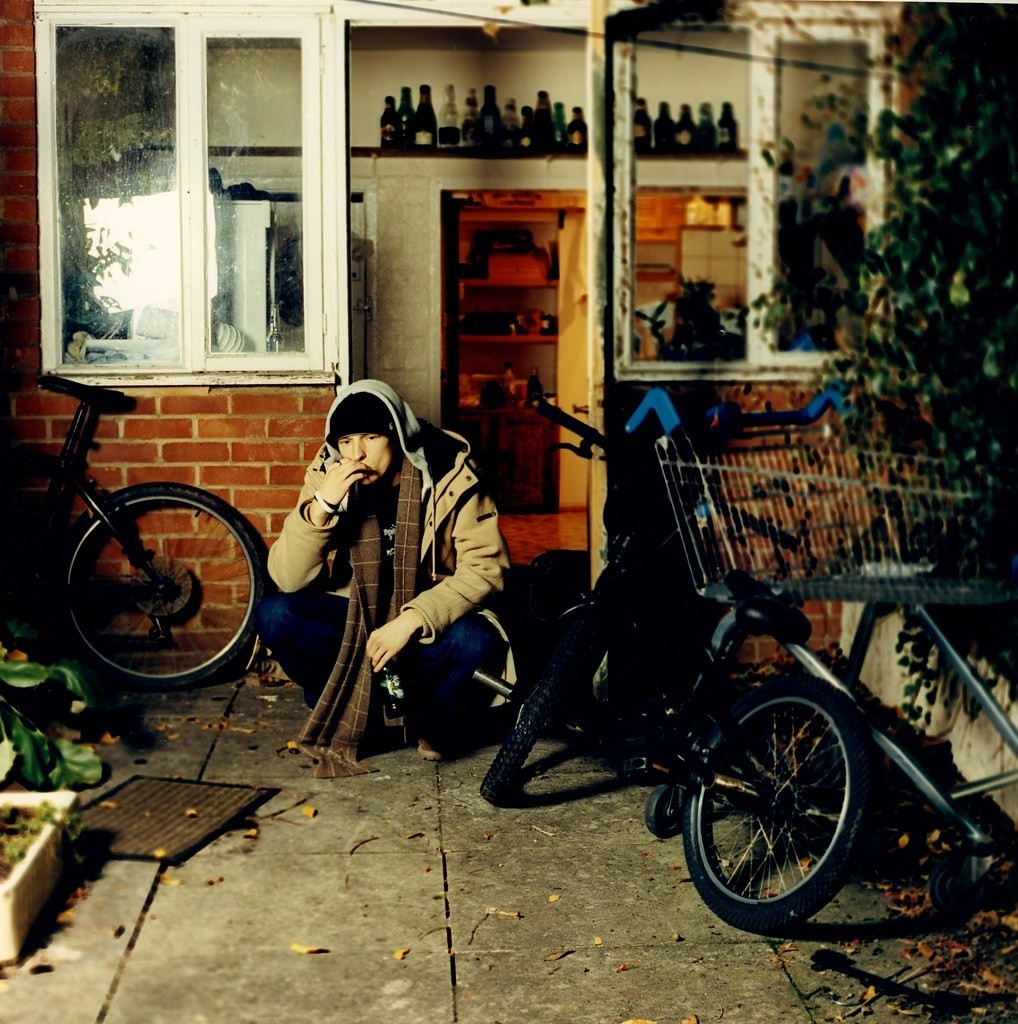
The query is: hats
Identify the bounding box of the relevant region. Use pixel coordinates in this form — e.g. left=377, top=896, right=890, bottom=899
left=326, top=392, right=398, bottom=454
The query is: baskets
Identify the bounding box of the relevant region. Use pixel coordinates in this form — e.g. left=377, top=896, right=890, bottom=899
left=658, top=422, right=1018, bottom=603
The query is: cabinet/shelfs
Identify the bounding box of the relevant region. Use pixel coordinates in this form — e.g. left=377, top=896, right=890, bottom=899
left=446, top=195, right=562, bottom=514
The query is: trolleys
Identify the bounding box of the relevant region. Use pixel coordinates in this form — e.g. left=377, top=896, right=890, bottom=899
left=625, top=387, right=1018, bottom=927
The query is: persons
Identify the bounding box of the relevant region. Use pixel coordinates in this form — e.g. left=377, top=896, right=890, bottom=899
left=252, top=378, right=518, bottom=780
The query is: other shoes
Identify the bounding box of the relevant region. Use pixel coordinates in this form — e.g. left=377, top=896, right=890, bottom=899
left=402, top=717, right=457, bottom=760
left=355, top=706, right=387, bottom=760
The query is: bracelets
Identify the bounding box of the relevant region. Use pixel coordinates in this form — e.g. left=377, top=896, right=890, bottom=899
left=313, top=490, right=341, bottom=514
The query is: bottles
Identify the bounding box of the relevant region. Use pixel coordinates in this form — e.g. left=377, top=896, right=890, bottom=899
left=381, top=82, right=587, bottom=154
left=266, top=304, right=286, bottom=352
left=526, top=367, right=542, bottom=409
left=633, top=97, right=736, bottom=156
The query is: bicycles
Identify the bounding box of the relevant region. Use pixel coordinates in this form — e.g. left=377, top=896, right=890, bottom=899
left=0, top=374, right=279, bottom=691
left=479, top=397, right=878, bottom=934
